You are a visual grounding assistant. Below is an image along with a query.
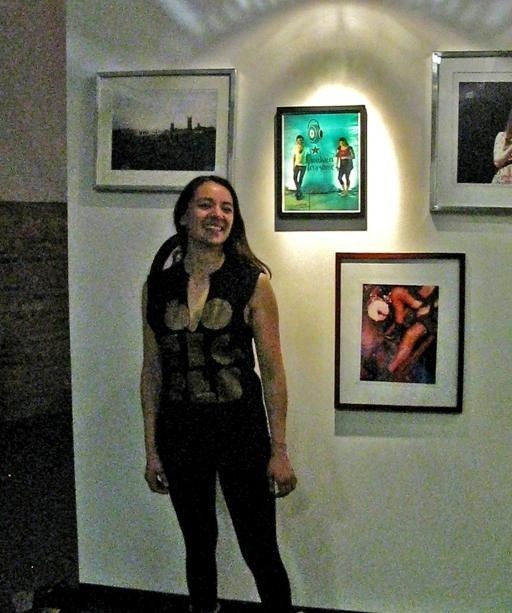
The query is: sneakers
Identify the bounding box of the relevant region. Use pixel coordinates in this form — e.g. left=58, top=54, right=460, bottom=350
left=374, top=368, right=394, bottom=382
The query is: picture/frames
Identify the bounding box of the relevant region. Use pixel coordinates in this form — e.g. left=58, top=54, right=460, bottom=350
left=93, top=68, right=237, bottom=194
left=428, top=52, right=512, bottom=215
left=275, top=106, right=368, bottom=221
left=334, top=252, right=466, bottom=414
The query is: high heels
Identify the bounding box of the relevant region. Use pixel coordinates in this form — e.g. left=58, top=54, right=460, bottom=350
left=386, top=321, right=406, bottom=341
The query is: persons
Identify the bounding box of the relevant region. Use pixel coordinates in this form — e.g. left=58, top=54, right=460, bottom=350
left=373, top=285, right=439, bottom=382
left=490, top=106, right=512, bottom=184
left=137, top=174, right=299, bottom=611
left=335, top=136, right=355, bottom=196
left=293, top=134, right=307, bottom=199
